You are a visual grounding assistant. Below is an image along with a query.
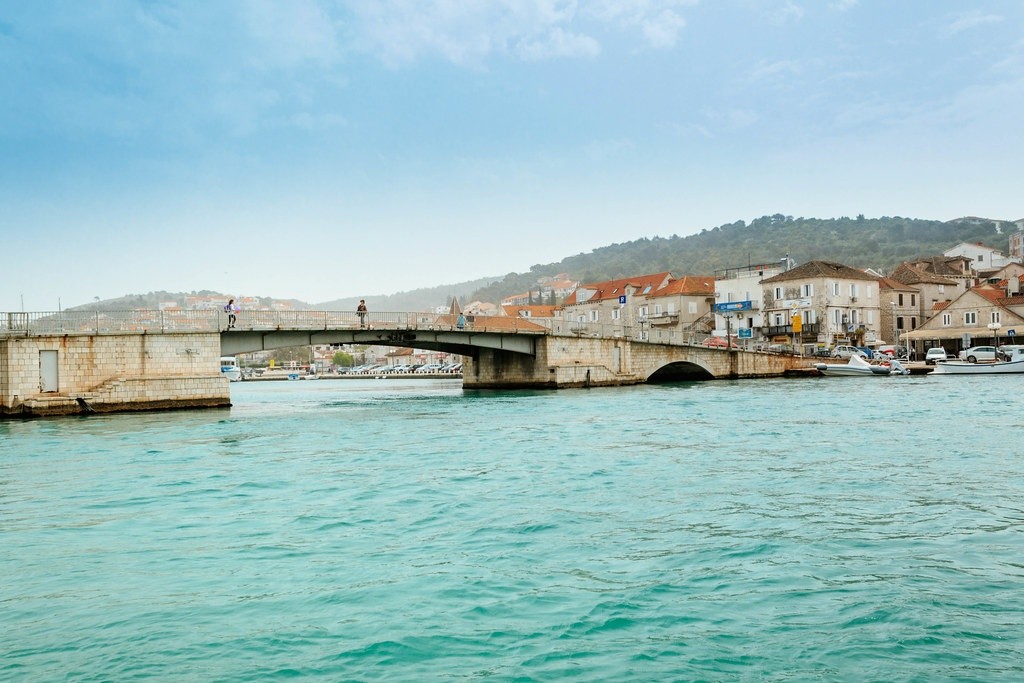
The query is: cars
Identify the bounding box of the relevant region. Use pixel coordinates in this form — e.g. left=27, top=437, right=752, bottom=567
left=701, top=335, right=738, bottom=349
left=337, top=362, right=463, bottom=375
left=857, top=347, right=874, bottom=360
left=872, top=350, right=892, bottom=360
left=998, top=345, right=1024, bottom=363
left=959, top=346, right=999, bottom=363
left=830, top=345, right=868, bottom=360
left=924, top=347, right=947, bottom=365
left=768, top=343, right=800, bottom=355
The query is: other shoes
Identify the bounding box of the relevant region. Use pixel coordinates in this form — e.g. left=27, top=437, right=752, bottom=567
left=228, top=325, right=231, bottom=328
left=232, top=325, right=235, bottom=328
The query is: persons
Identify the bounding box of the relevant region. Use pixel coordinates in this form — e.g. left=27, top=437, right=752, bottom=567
left=456, top=311, right=477, bottom=330
left=226, top=300, right=237, bottom=328
left=356, top=300, right=367, bottom=327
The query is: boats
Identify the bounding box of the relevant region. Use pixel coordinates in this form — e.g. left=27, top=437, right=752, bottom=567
left=220, top=357, right=242, bottom=383
left=813, top=354, right=911, bottom=375
left=811, top=350, right=832, bottom=357
left=243, top=365, right=321, bottom=380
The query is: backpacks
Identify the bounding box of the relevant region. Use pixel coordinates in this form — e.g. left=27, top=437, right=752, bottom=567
left=224, top=304, right=232, bottom=313
